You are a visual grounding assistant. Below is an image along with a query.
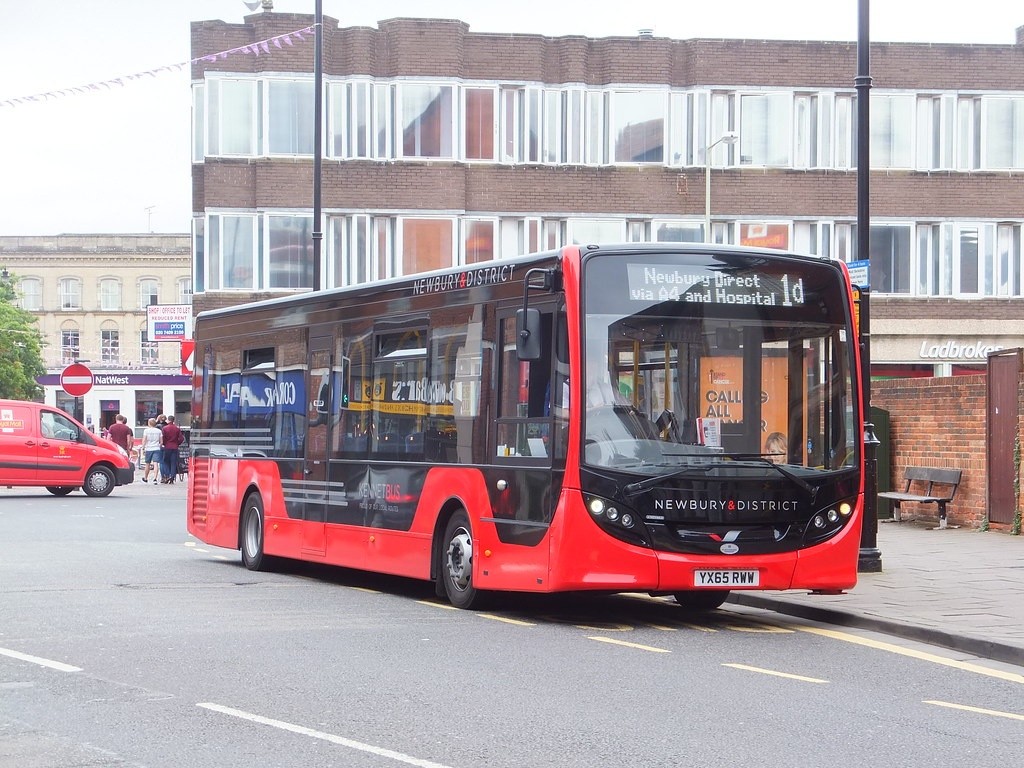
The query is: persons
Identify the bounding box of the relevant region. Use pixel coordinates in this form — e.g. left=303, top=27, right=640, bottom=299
left=763, top=432, right=788, bottom=465
left=102, top=413, right=184, bottom=485
left=41, top=420, right=54, bottom=439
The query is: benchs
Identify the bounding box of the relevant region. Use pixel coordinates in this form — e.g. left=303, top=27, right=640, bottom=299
left=877, top=466, right=963, bottom=531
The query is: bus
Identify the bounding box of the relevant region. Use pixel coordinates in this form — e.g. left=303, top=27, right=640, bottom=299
left=184, top=240, right=867, bottom=614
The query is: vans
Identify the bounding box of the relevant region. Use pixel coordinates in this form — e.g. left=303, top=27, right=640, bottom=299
left=0, top=399, right=137, bottom=497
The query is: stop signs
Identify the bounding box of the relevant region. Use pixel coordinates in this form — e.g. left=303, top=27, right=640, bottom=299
left=61, top=364, right=95, bottom=397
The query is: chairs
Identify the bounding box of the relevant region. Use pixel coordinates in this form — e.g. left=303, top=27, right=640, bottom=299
left=342, top=431, right=426, bottom=454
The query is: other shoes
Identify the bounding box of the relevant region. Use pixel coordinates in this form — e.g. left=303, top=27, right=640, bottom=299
left=153, top=478, right=157, bottom=485
left=142, top=477, right=148, bottom=483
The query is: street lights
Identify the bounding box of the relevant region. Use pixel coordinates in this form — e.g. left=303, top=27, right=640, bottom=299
left=702, top=133, right=740, bottom=245
